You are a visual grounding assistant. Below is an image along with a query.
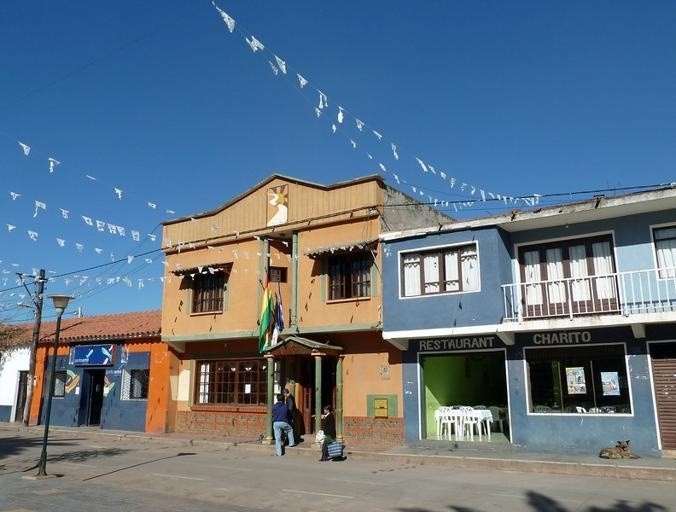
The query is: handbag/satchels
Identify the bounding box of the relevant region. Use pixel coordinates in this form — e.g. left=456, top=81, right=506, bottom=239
left=328, top=441, right=342, bottom=457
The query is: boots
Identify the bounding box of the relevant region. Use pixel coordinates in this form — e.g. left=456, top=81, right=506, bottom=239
left=320, top=456, right=326, bottom=461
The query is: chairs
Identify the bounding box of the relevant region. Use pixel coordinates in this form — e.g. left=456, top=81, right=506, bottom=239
left=434, top=404, right=507, bottom=442
left=533, top=404, right=617, bottom=413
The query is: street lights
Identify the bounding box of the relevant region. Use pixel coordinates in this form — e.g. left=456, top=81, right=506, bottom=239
left=37, top=294, right=74, bottom=475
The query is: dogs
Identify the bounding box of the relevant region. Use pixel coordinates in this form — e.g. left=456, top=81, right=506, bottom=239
left=598, top=439, right=641, bottom=459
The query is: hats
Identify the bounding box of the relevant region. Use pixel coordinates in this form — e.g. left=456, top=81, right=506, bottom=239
left=282, top=390, right=288, bottom=393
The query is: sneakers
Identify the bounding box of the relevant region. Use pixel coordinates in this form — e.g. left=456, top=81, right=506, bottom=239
left=291, top=442, right=297, bottom=447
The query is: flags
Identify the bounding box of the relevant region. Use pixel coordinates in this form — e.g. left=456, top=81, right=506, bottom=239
left=258, top=272, right=282, bottom=354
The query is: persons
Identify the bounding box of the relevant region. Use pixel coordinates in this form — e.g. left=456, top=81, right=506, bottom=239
left=272, top=393, right=296, bottom=456
left=283, top=389, right=301, bottom=445
left=319, top=404, right=336, bottom=461
